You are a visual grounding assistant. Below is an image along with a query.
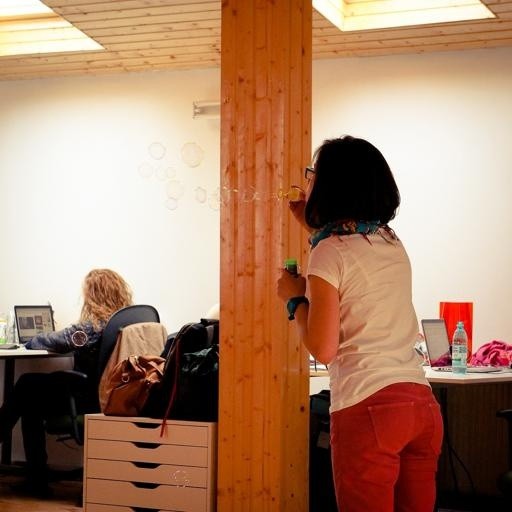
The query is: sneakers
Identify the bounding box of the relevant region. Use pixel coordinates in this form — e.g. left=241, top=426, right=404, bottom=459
left=8, top=479, right=50, bottom=493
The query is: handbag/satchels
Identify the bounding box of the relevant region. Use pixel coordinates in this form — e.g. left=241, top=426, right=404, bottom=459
left=159, top=316, right=219, bottom=376
left=152, top=348, right=219, bottom=424
left=102, top=356, right=165, bottom=416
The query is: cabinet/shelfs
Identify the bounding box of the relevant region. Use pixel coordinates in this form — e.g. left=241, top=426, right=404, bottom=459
left=81, top=411, right=218, bottom=512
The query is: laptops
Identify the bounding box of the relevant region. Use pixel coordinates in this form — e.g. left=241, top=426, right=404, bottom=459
left=15, top=306, right=55, bottom=345
left=421, top=319, right=502, bottom=373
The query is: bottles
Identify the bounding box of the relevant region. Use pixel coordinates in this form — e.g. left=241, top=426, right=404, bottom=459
left=452, top=323, right=468, bottom=375
left=1, top=307, right=16, bottom=343
left=283, top=258, right=298, bottom=276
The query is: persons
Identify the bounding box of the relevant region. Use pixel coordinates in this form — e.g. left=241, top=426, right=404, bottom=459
left=277, top=135, right=446, bottom=512
left=1, top=268, right=133, bottom=498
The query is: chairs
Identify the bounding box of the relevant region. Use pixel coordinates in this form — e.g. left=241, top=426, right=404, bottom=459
left=42, top=302, right=162, bottom=508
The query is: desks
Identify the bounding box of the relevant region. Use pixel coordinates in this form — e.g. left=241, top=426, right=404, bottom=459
left=0, top=343, right=76, bottom=473
left=423, top=364, right=512, bottom=512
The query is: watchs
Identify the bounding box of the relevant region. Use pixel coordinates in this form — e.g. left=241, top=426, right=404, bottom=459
left=286, top=296, right=309, bottom=320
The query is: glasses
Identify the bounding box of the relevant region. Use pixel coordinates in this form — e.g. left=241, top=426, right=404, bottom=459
left=304, top=165, right=319, bottom=180
